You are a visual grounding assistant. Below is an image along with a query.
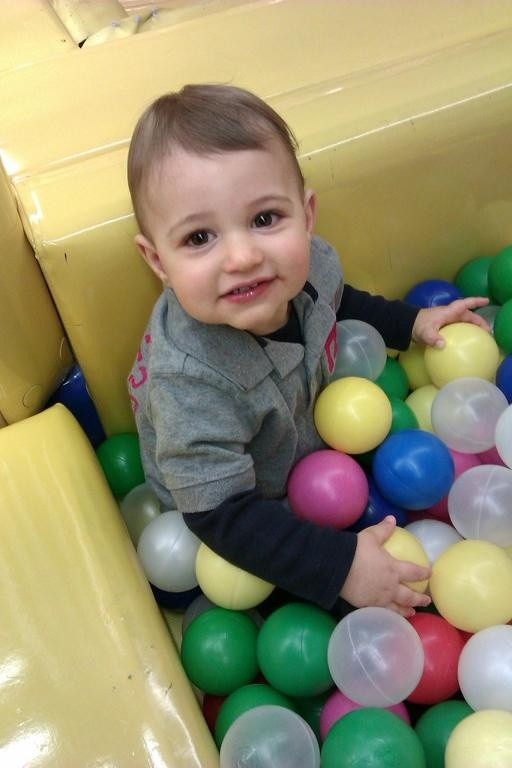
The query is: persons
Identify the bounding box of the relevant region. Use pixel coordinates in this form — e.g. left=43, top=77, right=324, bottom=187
left=123, top=79, right=493, bottom=622
